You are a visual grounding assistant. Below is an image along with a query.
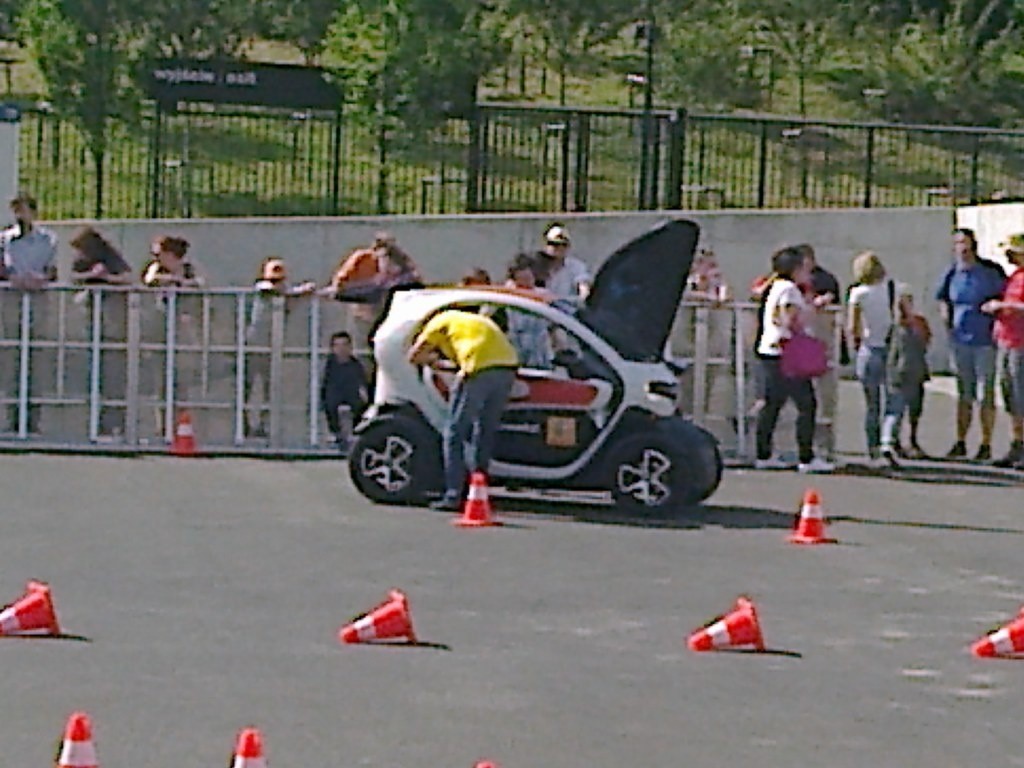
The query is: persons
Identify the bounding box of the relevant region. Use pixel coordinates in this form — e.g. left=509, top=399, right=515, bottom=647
left=0, top=196, right=59, bottom=436
left=542, top=223, right=590, bottom=352
left=933, top=229, right=1008, bottom=464
left=980, top=233, right=1024, bottom=471
left=333, top=233, right=398, bottom=381
left=670, top=248, right=730, bottom=424
left=138, top=236, right=232, bottom=435
left=460, top=253, right=554, bottom=369
left=232, top=257, right=316, bottom=438
left=320, top=331, right=369, bottom=443
left=893, top=283, right=932, bottom=459
left=68, top=226, right=134, bottom=400
left=736, top=239, right=839, bottom=472
left=409, top=307, right=518, bottom=510
left=846, top=251, right=904, bottom=470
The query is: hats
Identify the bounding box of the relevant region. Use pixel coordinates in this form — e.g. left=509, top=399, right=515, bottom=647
left=999, top=234, right=1024, bottom=253
left=546, top=226, right=572, bottom=244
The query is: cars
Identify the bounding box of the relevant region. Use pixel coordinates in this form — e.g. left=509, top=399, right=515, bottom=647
left=332, top=281, right=723, bottom=512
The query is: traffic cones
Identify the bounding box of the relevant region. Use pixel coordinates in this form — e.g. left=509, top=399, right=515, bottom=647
left=686, top=595, right=764, bottom=655
left=231, top=726, right=270, bottom=768
left=449, top=468, right=500, bottom=528
left=339, top=587, right=419, bottom=646
left=170, top=409, right=202, bottom=456
left=53, top=710, right=102, bottom=768
left=784, top=487, right=842, bottom=543
left=0, top=581, right=60, bottom=639
left=972, top=605, right=1024, bottom=659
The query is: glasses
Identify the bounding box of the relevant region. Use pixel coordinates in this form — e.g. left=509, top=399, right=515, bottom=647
left=549, top=242, right=567, bottom=247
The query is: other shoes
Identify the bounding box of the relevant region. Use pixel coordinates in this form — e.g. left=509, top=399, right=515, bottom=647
left=908, top=444, right=924, bottom=459
left=798, top=456, right=836, bottom=472
left=880, top=445, right=901, bottom=468
left=869, top=449, right=886, bottom=469
left=756, top=456, right=789, bottom=470
left=888, top=442, right=906, bottom=460
left=993, top=445, right=1013, bottom=468
left=429, top=495, right=462, bottom=511
left=973, top=443, right=993, bottom=464
left=946, top=442, right=967, bottom=462
left=1013, top=446, right=1024, bottom=469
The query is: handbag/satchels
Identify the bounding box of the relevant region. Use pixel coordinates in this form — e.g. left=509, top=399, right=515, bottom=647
left=839, top=337, right=850, bottom=365
left=782, top=310, right=827, bottom=377
